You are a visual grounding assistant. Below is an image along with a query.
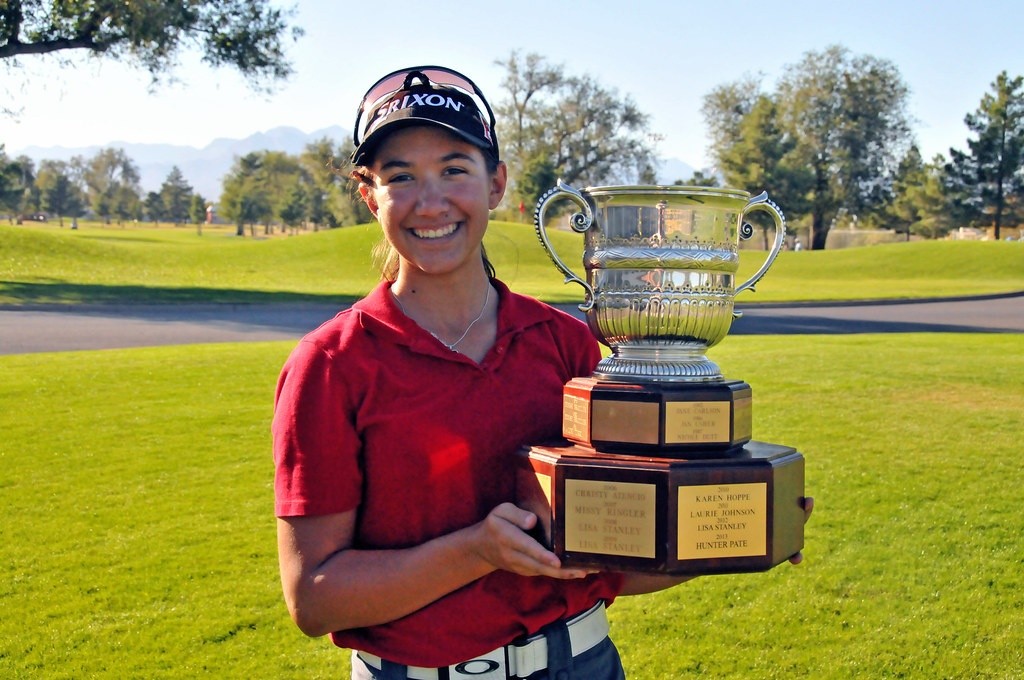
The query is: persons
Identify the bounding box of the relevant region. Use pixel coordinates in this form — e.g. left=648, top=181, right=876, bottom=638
left=271, top=65, right=814, bottom=680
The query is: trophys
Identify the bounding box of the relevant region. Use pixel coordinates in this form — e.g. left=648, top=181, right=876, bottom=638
left=514, top=178, right=805, bottom=575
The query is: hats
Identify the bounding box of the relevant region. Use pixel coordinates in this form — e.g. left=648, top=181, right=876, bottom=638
left=351, top=84, right=500, bottom=164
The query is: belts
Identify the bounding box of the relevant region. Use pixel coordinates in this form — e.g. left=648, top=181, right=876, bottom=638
left=356, top=600, right=609, bottom=680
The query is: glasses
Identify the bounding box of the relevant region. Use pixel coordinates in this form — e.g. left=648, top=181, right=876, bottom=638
left=353, top=66, right=496, bottom=147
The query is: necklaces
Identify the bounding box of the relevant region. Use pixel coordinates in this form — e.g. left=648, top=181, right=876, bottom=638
left=392, top=282, right=490, bottom=354
